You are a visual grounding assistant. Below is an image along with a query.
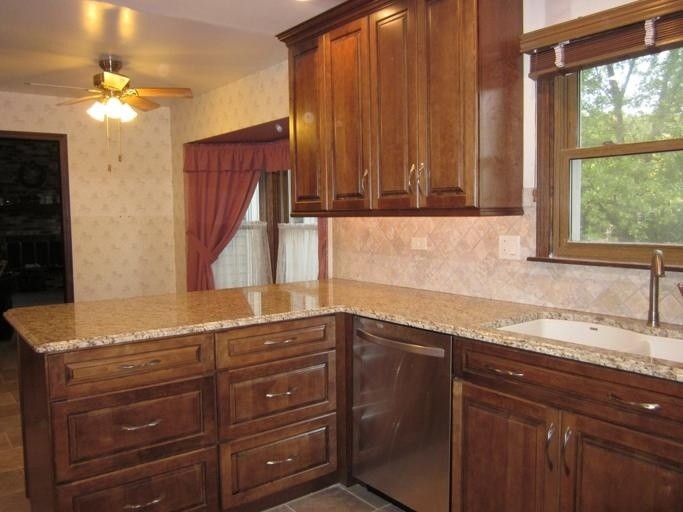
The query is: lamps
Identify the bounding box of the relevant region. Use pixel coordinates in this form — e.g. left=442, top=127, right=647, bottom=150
left=87, top=96, right=137, bottom=124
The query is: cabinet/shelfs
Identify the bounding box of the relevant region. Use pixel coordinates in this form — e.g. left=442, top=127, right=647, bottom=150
left=451, top=333, right=683, bottom=512
left=17, top=332, right=215, bottom=512
left=215, top=313, right=347, bottom=511
left=366, top=1, right=525, bottom=216
left=288, top=14, right=371, bottom=216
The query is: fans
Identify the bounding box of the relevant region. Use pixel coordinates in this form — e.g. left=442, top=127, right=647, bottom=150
left=22, top=53, right=193, bottom=111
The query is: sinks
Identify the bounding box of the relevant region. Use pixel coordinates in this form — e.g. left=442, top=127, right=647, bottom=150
left=488, top=313, right=679, bottom=370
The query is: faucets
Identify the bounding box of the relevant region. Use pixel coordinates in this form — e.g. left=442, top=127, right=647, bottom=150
left=649, top=249, right=664, bottom=329
